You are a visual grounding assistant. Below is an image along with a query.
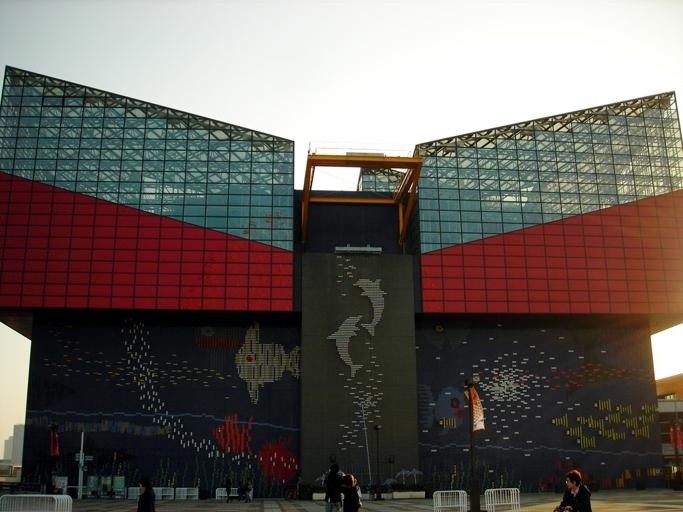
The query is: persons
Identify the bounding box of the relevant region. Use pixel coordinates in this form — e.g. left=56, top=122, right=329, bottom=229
left=237, top=477, right=251, bottom=503
left=226, top=479, right=232, bottom=503
left=324, top=464, right=362, bottom=512
left=553, top=470, right=592, bottom=512
left=137, top=482, right=155, bottom=512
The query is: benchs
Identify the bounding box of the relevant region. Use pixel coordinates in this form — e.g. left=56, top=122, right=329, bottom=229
left=126, top=485, right=198, bottom=501
left=215, top=486, right=253, bottom=501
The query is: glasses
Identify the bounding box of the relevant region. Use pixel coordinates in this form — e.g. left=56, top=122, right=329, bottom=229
left=565, top=480, right=571, bottom=484
